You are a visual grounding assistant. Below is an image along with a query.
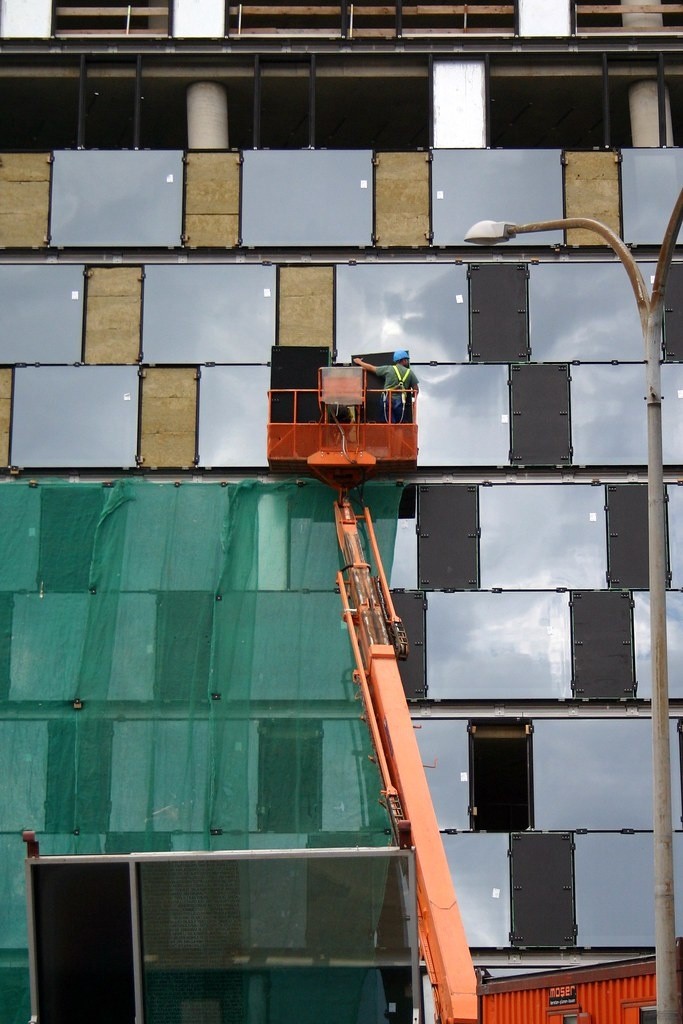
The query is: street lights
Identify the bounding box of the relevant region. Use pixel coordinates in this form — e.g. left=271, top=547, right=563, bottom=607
left=464, top=215, right=682, bottom=1024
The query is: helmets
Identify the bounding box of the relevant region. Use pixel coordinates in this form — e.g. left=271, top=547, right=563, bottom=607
left=393, top=350, right=410, bottom=362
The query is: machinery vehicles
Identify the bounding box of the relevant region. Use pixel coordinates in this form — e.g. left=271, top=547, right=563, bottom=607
left=268, top=363, right=683, bottom=1024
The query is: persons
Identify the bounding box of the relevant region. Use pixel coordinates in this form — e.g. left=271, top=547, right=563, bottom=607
left=354, top=350, right=419, bottom=423
